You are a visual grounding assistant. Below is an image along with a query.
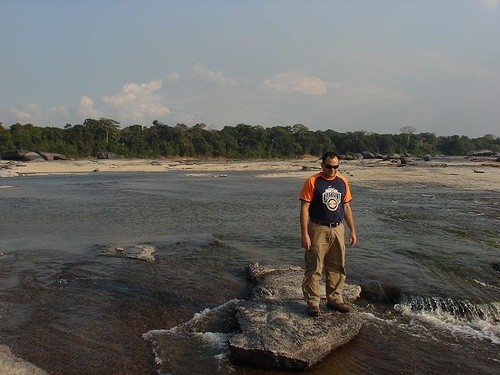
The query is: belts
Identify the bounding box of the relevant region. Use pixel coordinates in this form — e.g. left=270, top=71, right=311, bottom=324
left=310, top=218, right=342, bottom=228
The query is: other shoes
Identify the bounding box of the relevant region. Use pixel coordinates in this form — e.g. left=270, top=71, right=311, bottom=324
left=307, top=305, right=320, bottom=316
left=327, top=302, right=350, bottom=313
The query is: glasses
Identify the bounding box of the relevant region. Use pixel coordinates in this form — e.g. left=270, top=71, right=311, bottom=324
left=324, top=163, right=339, bottom=169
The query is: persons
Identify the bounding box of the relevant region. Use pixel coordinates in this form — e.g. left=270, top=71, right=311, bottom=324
left=397, top=155, right=407, bottom=168
left=297, top=152, right=357, bottom=316
